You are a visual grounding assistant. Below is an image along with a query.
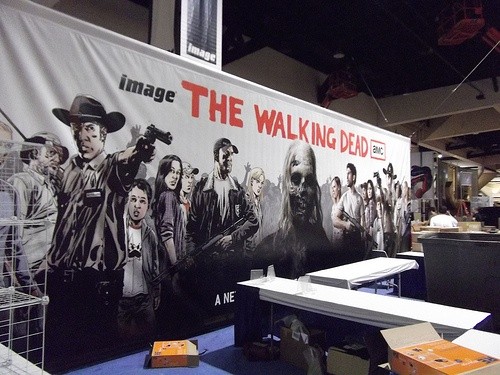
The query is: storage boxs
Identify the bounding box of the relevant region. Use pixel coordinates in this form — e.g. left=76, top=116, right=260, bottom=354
left=377, top=322, right=500, bottom=375
left=411, top=221, right=481, bottom=251
left=145, top=339, right=199, bottom=369
left=327, top=336, right=374, bottom=375
left=280, top=326, right=329, bottom=369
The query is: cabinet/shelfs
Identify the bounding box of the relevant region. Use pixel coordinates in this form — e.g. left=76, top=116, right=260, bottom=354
left=0, top=139, right=52, bottom=375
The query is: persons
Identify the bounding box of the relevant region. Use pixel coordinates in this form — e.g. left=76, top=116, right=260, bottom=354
left=430, top=205, right=458, bottom=227
left=427, top=206, right=437, bottom=226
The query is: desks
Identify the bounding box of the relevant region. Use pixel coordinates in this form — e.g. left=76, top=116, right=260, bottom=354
left=234, top=276, right=491, bottom=360
left=305, top=257, right=419, bottom=298
